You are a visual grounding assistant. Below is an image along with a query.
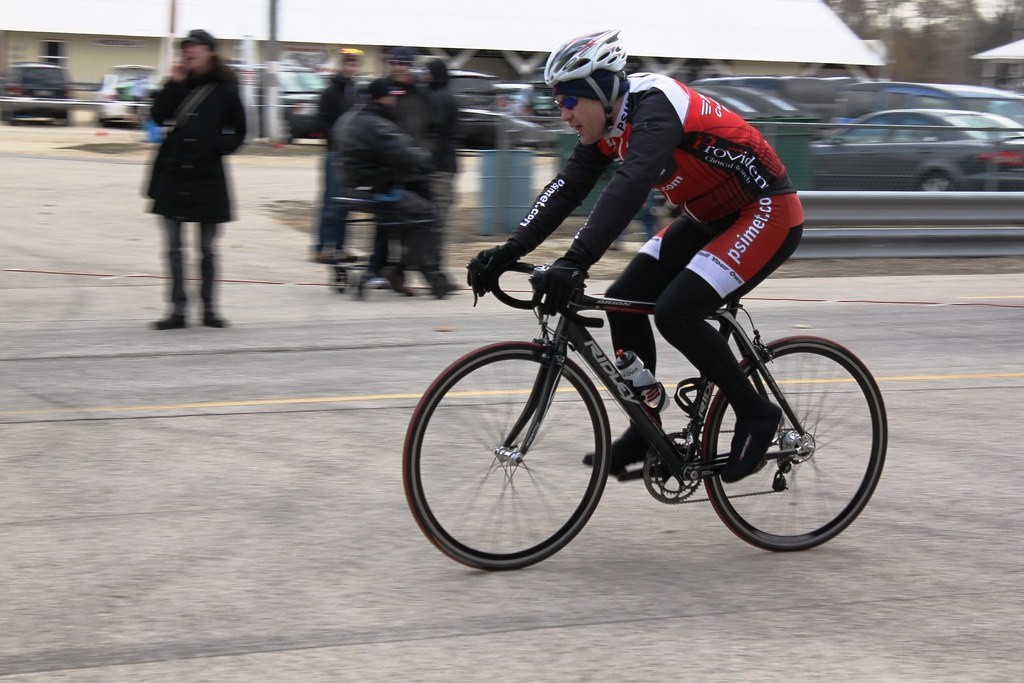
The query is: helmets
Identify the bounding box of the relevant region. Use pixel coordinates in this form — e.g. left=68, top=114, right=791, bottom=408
left=338, top=48, right=365, bottom=62
left=544, top=29, right=628, bottom=87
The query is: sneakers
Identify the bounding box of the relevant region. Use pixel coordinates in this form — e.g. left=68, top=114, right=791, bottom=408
left=722, top=397, right=781, bottom=483
left=583, top=426, right=665, bottom=468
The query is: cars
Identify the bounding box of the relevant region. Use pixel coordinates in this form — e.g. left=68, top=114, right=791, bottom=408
left=98, top=64, right=162, bottom=126
left=689, top=77, right=1023, bottom=128
left=0, top=64, right=70, bottom=123
left=810, top=109, right=1024, bottom=192
left=228, top=58, right=558, bottom=150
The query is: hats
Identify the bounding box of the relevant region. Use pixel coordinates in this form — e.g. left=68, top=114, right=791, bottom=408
left=180, top=29, right=217, bottom=47
left=387, top=49, right=413, bottom=66
left=369, top=79, right=406, bottom=99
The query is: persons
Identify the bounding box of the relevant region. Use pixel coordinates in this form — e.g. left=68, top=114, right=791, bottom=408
left=316, top=47, right=459, bottom=301
left=147, top=28, right=247, bottom=330
left=467, top=26, right=805, bottom=484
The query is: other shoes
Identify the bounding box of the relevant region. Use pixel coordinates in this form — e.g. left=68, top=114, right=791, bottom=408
left=156, top=315, right=185, bottom=328
left=204, top=313, right=224, bottom=328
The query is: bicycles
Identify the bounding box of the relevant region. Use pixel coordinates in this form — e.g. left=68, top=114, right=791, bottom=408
left=402, top=257, right=889, bottom=572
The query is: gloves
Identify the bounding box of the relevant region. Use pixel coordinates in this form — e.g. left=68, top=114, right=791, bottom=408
left=532, top=258, right=589, bottom=315
left=468, top=245, right=523, bottom=298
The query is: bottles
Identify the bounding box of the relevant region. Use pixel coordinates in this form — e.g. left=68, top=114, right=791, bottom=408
left=616, top=350, right=670, bottom=413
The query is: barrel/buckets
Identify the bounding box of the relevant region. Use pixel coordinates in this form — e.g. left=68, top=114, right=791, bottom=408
left=478, top=149, right=535, bottom=235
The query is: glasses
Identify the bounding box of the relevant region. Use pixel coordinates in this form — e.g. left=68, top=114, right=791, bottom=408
left=554, top=96, right=577, bottom=110
